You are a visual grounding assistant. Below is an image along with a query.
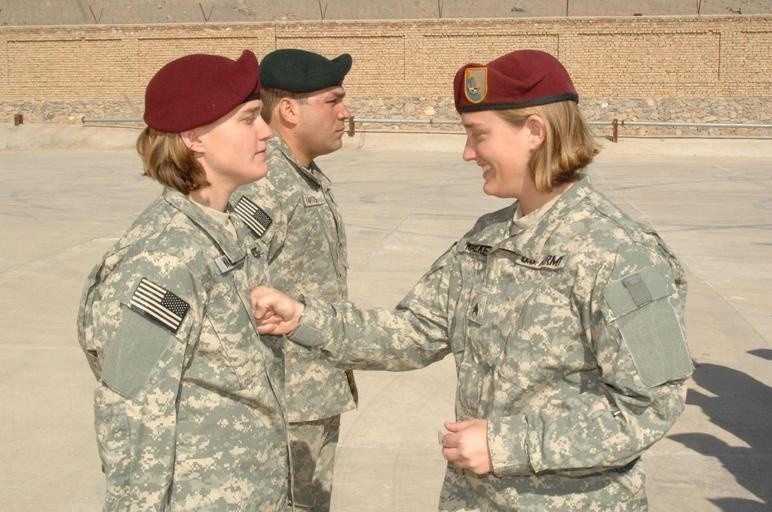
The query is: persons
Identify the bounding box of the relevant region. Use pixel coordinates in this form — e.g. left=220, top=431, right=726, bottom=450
left=80, top=48, right=296, bottom=512
left=250, top=50, right=694, bottom=512
left=230, top=49, right=358, bottom=512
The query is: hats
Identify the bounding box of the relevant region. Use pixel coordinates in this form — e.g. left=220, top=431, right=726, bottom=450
left=259, top=49, right=352, bottom=94
left=142, top=49, right=262, bottom=134
left=453, top=49, right=579, bottom=115
